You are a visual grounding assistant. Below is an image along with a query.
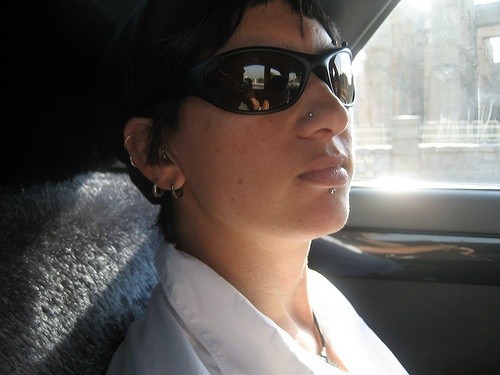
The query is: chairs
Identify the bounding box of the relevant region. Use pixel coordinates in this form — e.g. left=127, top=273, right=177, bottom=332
left=0, top=170, right=161, bottom=375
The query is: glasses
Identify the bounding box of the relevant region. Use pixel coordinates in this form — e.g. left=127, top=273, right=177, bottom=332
left=156, top=46, right=357, bottom=116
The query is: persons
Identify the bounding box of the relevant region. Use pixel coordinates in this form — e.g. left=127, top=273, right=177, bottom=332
left=96, top=0, right=410, bottom=375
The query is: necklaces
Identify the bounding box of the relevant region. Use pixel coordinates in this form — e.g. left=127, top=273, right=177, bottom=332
left=313, top=313, right=331, bottom=364
